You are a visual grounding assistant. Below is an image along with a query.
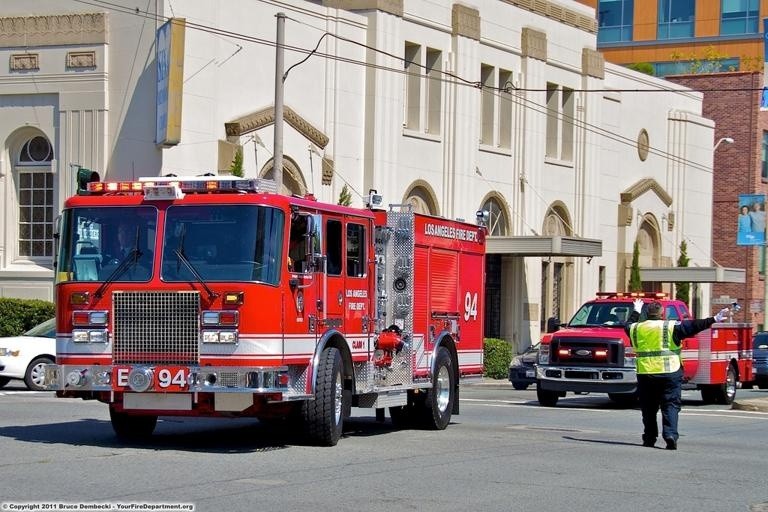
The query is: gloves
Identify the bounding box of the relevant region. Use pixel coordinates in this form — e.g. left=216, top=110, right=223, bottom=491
left=714, top=308, right=729, bottom=322
left=633, top=299, right=644, bottom=313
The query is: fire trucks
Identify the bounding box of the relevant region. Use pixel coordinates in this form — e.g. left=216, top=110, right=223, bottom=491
left=535, top=291, right=758, bottom=408
left=53, top=165, right=487, bottom=448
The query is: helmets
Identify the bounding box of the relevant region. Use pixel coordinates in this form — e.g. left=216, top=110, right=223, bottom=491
left=648, top=301, right=664, bottom=316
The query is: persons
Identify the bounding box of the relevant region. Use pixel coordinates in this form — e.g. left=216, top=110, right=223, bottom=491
left=738, top=202, right=766, bottom=232
left=112, top=223, right=145, bottom=267
left=624, top=297, right=729, bottom=450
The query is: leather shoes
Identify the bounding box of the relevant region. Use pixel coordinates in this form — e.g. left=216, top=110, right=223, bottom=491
left=643, top=436, right=677, bottom=450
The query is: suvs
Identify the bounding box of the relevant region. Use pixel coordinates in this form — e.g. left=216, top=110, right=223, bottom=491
left=743, top=330, right=768, bottom=390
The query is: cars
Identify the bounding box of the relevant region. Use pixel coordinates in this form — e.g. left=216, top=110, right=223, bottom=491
left=0, top=314, right=56, bottom=395
left=507, top=339, right=590, bottom=396
